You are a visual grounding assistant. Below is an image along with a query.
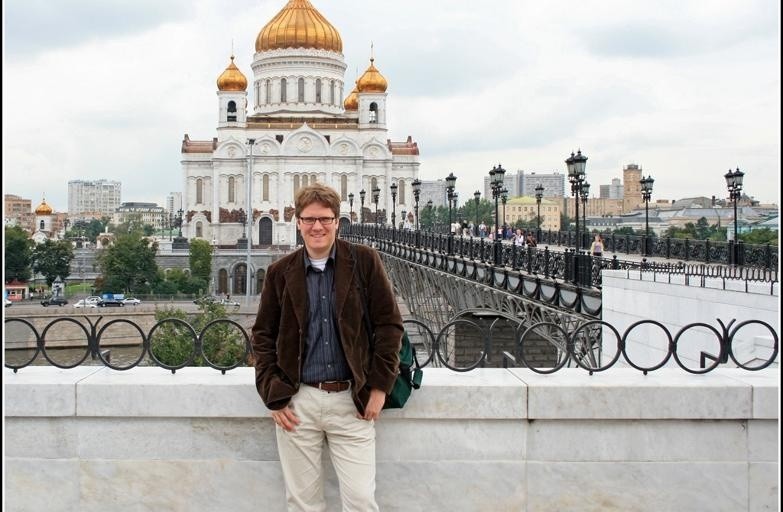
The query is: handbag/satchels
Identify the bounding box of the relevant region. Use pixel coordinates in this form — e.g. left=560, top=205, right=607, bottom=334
left=369, top=330, right=423, bottom=408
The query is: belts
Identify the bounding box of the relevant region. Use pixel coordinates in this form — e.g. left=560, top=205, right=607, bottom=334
left=309, top=381, right=352, bottom=391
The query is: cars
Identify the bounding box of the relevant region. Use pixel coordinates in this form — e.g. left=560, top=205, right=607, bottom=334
left=39, top=295, right=67, bottom=308
left=71, top=295, right=141, bottom=308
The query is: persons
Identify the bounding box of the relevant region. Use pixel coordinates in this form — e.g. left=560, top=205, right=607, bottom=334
left=451, top=219, right=537, bottom=247
left=252, top=183, right=402, bottom=512
left=590, top=234, right=604, bottom=256
left=29, top=290, right=33, bottom=301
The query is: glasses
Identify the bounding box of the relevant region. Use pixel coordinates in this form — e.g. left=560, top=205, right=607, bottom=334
left=299, top=216, right=336, bottom=224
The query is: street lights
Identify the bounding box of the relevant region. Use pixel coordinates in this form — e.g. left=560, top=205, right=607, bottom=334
left=638, top=174, right=655, bottom=256
left=388, top=183, right=398, bottom=243
left=348, top=192, right=353, bottom=233
left=243, top=137, right=258, bottom=298
left=359, top=188, right=366, bottom=234
left=487, top=163, right=505, bottom=243
left=723, top=167, right=744, bottom=267
left=562, top=149, right=588, bottom=252
left=444, top=171, right=457, bottom=236
left=399, top=179, right=595, bottom=247
left=371, top=186, right=382, bottom=236
